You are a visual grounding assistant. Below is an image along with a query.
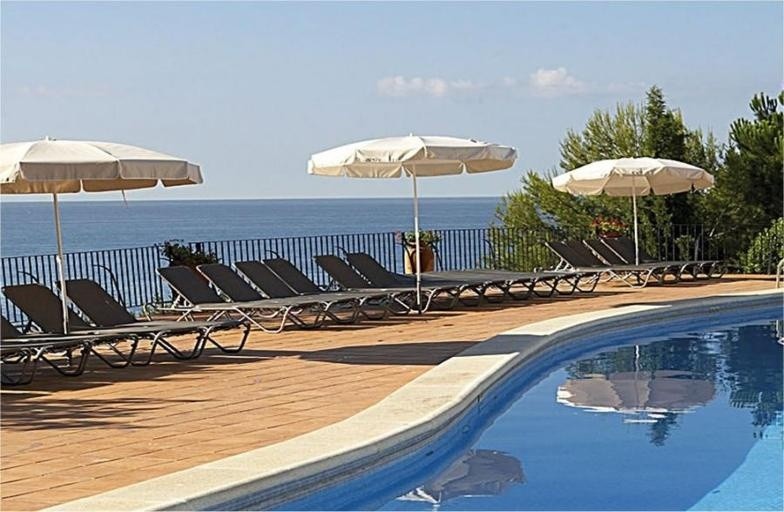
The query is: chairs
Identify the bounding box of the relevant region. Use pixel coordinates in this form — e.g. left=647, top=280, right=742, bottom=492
left=154, top=258, right=416, bottom=334
left=1, top=277, right=251, bottom=388
left=310, top=252, right=610, bottom=314
left=534, top=239, right=729, bottom=289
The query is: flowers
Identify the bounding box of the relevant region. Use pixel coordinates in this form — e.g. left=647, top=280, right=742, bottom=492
left=589, top=214, right=628, bottom=235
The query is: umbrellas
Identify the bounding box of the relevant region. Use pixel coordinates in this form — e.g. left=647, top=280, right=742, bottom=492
left=0, top=135, right=207, bottom=367
left=304, top=128, right=521, bottom=316
left=549, top=155, right=717, bottom=287
left=396, top=447, right=529, bottom=511
left=551, top=344, right=719, bottom=432
